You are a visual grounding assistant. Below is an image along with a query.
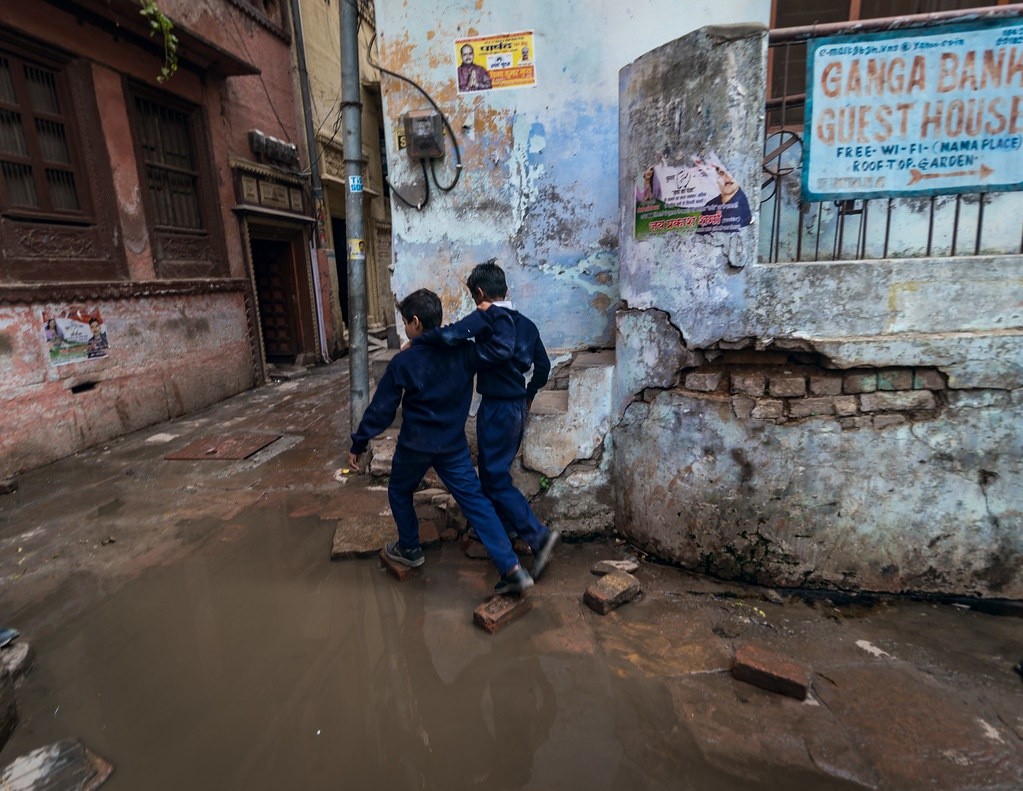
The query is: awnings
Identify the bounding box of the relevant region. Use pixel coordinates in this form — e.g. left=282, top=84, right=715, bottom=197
left=72, top=0, right=262, bottom=78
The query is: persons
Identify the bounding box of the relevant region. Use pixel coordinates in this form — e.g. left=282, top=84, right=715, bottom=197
left=87, top=318, right=108, bottom=358
left=636, top=168, right=662, bottom=202
left=347, top=288, right=534, bottom=594
left=517, top=47, right=533, bottom=67
left=374, top=582, right=558, bottom=791
left=357, top=241, right=365, bottom=260
left=457, top=44, right=492, bottom=92
left=400, top=257, right=560, bottom=578
left=45, top=319, right=63, bottom=340
left=696, top=167, right=752, bottom=235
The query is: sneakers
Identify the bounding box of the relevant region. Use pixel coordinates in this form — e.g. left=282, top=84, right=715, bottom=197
left=493, top=567, right=534, bottom=594
left=527, top=529, right=558, bottom=578
left=467, top=525, right=518, bottom=539
left=385, top=539, right=425, bottom=567
